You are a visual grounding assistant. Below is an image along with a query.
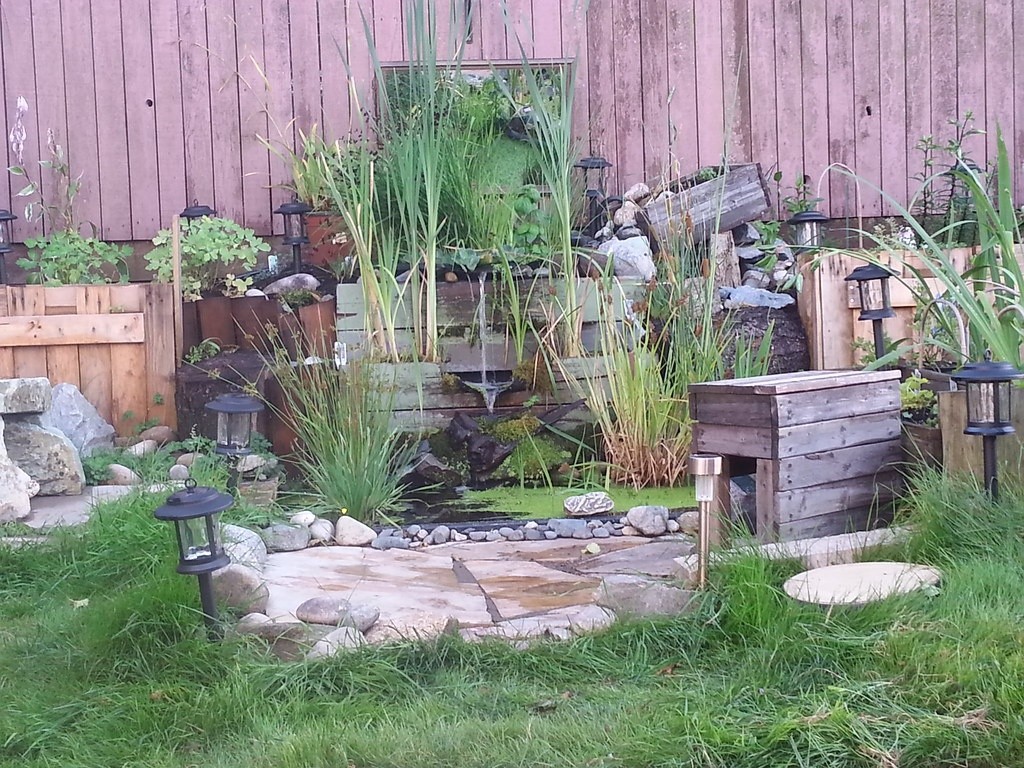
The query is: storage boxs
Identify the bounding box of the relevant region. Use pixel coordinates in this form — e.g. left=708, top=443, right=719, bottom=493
left=609, top=163, right=771, bottom=254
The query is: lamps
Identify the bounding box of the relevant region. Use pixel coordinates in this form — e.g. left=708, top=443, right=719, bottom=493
left=154, top=476, right=234, bottom=642
left=204, top=381, right=265, bottom=497
left=573, top=150, right=613, bottom=239
left=0, top=209, right=18, bottom=284
left=951, top=349, right=1020, bottom=503
left=274, top=195, right=312, bottom=274
left=785, top=204, right=830, bottom=253
left=179, top=199, right=218, bottom=227
left=844, top=260, right=901, bottom=372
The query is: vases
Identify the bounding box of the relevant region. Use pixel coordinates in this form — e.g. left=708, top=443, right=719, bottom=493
left=182, top=301, right=202, bottom=353
left=231, top=295, right=282, bottom=351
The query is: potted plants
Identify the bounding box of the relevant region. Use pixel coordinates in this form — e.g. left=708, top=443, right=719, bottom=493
left=278, top=290, right=336, bottom=361
left=785, top=116, right=1024, bottom=488
left=143, top=213, right=271, bottom=353
left=254, top=123, right=354, bottom=265
